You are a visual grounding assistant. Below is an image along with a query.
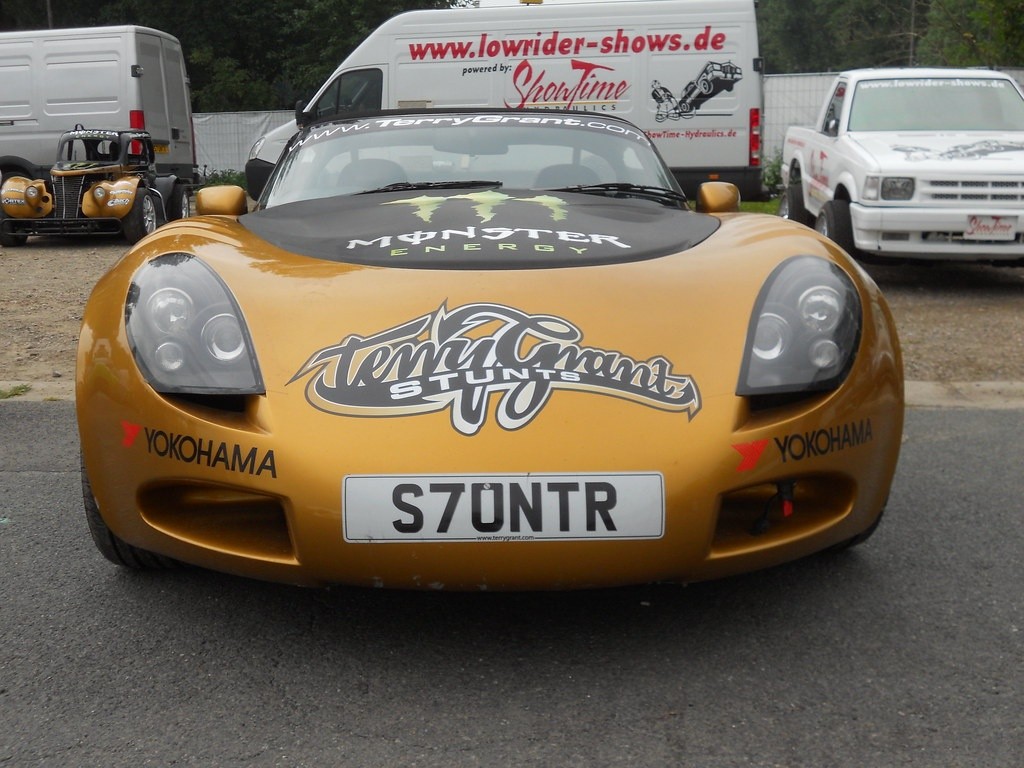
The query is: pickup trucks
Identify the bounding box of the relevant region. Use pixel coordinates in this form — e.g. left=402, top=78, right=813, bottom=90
left=776, top=67, right=1024, bottom=271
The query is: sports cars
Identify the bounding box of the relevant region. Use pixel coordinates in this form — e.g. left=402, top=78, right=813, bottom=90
left=72, top=102, right=908, bottom=613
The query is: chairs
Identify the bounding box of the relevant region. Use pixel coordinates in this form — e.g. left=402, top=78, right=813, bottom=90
left=337, top=159, right=407, bottom=193
left=537, top=164, right=602, bottom=190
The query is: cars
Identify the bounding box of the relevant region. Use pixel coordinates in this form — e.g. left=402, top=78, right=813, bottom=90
left=0, top=124, right=190, bottom=246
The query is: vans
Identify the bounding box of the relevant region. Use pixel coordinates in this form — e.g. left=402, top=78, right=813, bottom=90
left=244, top=0, right=777, bottom=205
left=0, top=25, right=207, bottom=196
